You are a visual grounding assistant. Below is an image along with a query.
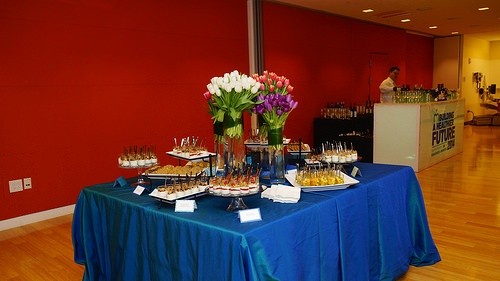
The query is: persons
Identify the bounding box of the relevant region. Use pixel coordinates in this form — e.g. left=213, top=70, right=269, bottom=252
left=379, top=67, right=400, bottom=103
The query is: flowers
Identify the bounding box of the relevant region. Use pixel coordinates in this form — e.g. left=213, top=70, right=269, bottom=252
left=203, top=70, right=298, bottom=145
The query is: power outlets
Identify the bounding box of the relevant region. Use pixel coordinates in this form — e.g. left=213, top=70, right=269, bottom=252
left=24, top=178, right=32, bottom=189
left=8, top=179, right=23, bottom=193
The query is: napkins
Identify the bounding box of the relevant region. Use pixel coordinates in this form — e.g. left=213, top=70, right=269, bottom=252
left=260, top=184, right=301, bottom=204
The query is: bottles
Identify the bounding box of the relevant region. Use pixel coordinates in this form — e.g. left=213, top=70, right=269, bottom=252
left=324, top=102, right=373, bottom=118
left=393, top=84, right=431, bottom=103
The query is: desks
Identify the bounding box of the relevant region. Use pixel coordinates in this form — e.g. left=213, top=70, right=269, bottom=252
left=70, top=152, right=442, bottom=281
